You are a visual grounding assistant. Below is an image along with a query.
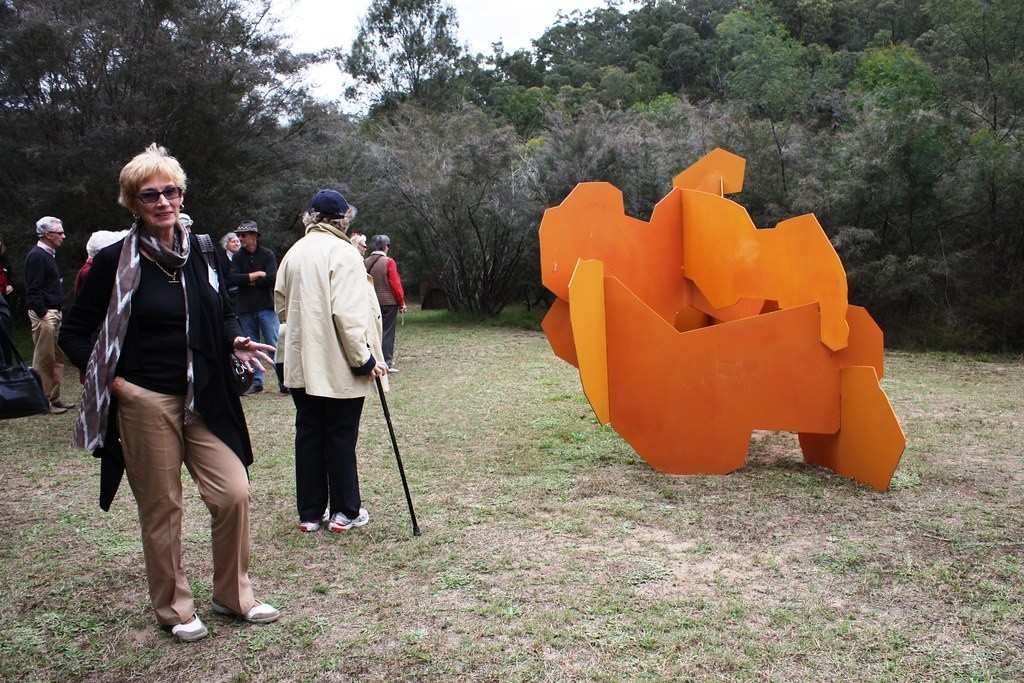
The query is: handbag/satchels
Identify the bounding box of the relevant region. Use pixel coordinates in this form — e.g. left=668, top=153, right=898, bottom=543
left=230, top=353, right=253, bottom=396
left=0, top=333, right=49, bottom=419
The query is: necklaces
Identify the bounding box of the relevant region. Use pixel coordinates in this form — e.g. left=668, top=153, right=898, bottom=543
left=158, top=263, right=180, bottom=283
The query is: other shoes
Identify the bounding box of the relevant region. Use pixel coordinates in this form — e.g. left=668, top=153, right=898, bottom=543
left=388, top=361, right=399, bottom=372
left=245, top=385, right=263, bottom=394
left=279, top=388, right=292, bottom=395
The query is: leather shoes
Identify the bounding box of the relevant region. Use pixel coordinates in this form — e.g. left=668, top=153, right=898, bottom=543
left=49, top=400, right=75, bottom=414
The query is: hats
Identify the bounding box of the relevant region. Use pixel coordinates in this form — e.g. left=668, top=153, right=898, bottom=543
left=233, top=219, right=262, bottom=237
left=178, top=213, right=193, bottom=227
left=307, top=188, right=358, bottom=218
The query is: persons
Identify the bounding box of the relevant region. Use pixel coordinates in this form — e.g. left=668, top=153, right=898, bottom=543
left=274, top=189, right=390, bottom=534
left=58, top=148, right=280, bottom=642
left=179, top=213, right=289, bottom=394
left=75, top=230, right=132, bottom=385
left=349, top=230, right=408, bottom=374
left=24, top=216, right=75, bottom=414
left=0, top=242, right=14, bottom=368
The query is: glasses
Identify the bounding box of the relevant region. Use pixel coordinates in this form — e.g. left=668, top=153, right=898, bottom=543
left=133, top=186, right=182, bottom=203
left=385, top=243, right=390, bottom=248
left=359, top=243, right=367, bottom=249
left=50, top=231, right=67, bottom=235
left=236, top=233, right=250, bottom=238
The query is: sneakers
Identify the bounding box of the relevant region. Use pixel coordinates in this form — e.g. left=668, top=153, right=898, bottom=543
left=167, top=612, right=208, bottom=641
left=299, top=508, right=329, bottom=531
left=328, top=508, right=369, bottom=533
left=212, top=596, right=280, bottom=622
